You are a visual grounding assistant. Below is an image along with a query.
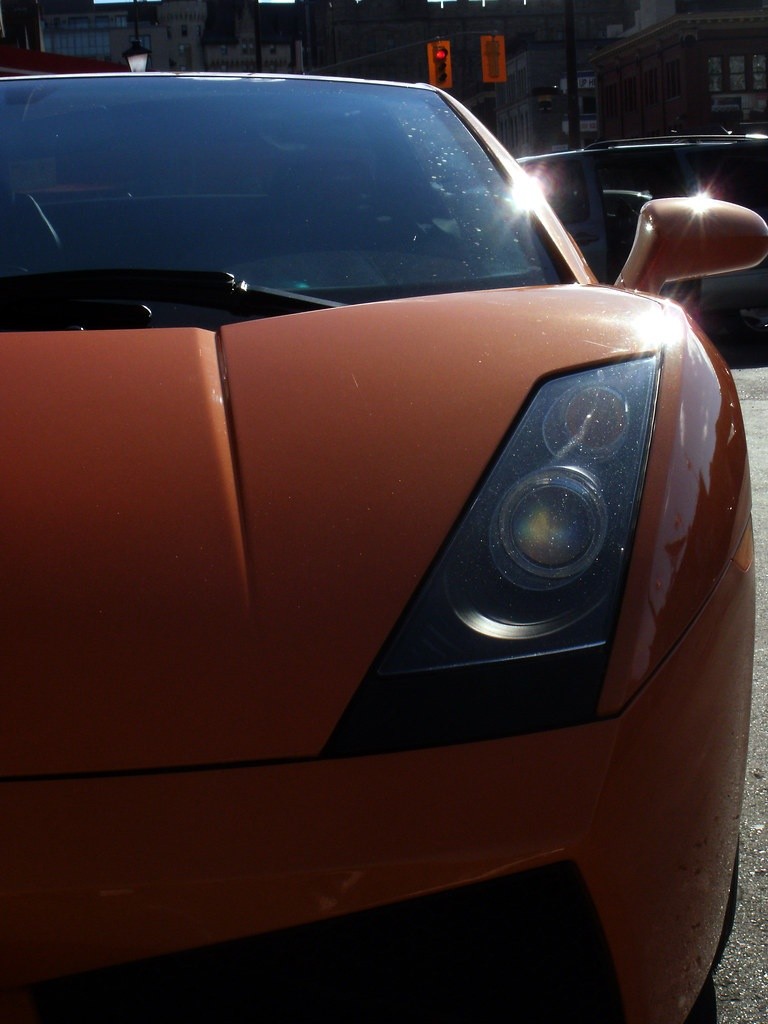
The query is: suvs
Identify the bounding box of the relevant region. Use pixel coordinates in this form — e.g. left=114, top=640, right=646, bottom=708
left=515, top=130, right=766, bottom=319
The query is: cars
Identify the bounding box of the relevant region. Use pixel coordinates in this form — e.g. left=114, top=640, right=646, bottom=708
left=0, top=68, right=768, bottom=1024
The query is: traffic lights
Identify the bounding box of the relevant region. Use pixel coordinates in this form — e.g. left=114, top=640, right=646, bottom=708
left=428, top=39, right=453, bottom=89
